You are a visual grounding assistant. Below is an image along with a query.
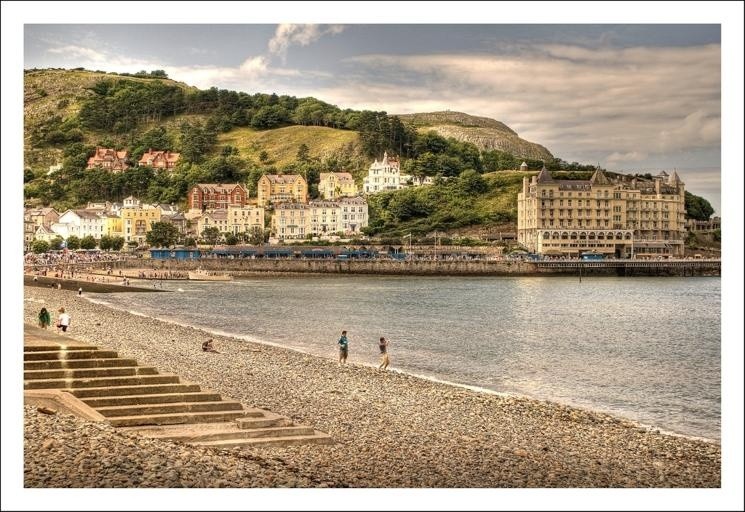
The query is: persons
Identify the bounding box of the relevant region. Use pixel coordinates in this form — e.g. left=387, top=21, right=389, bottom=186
left=202, top=339, right=221, bottom=354
left=379, top=337, right=390, bottom=370
left=337, top=330, right=348, bottom=364
left=57, top=307, right=72, bottom=335
left=38, top=308, right=50, bottom=330
left=24, top=251, right=189, bottom=297
